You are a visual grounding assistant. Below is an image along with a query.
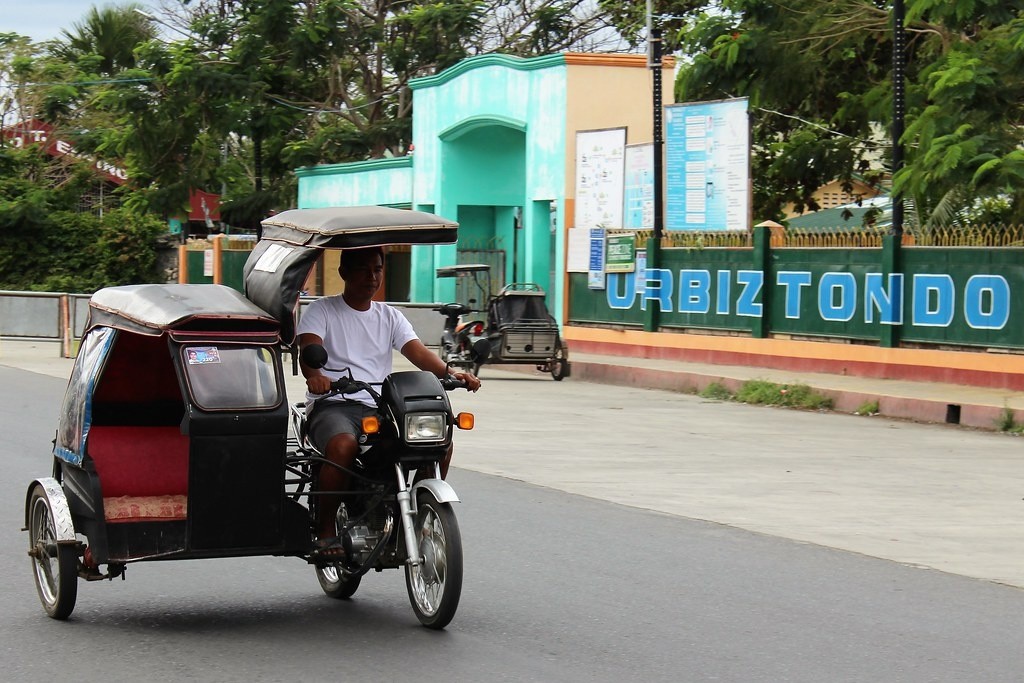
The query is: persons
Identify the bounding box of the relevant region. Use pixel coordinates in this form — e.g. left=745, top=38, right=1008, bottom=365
left=188, top=350, right=201, bottom=364
left=296, top=245, right=481, bottom=559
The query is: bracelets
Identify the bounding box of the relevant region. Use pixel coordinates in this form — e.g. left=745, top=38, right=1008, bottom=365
left=452, top=372, right=463, bottom=376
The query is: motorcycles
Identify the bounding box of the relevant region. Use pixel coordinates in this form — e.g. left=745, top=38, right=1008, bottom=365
left=432, top=265, right=567, bottom=381
left=22, top=207, right=477, bottom=628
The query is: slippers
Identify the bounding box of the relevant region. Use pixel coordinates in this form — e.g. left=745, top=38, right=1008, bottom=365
left=313, top=538, right=345, bottom=558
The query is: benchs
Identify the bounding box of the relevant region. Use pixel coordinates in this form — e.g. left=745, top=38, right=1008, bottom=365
left=69, top=424, right=190, bottom=555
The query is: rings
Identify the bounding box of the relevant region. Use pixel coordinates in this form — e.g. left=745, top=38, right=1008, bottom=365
left=475, top=377, right=479, bottom=380
